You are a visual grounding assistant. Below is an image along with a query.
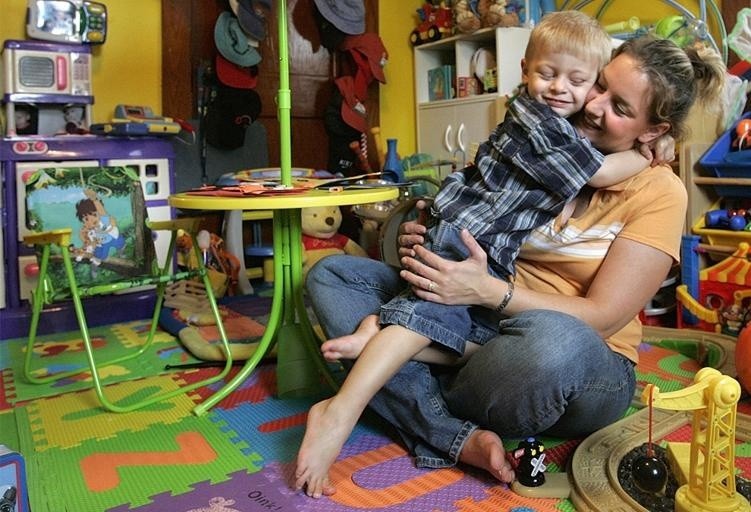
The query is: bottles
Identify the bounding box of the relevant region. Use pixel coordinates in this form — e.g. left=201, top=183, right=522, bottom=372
left=383, top=138, right=404, bottom=184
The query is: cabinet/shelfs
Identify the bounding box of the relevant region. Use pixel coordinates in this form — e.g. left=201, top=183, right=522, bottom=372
left=411, top=26, right=531, bottom=171
left=655, top=77, right=751, bottom=270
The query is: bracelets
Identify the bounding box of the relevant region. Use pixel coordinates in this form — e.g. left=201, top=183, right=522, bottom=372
left=494, top=283, right=514, bottom=311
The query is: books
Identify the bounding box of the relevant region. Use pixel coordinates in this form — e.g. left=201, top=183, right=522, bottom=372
left=427, top=65, right=456, bottom=101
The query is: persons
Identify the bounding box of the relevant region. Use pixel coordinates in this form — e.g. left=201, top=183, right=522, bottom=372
left=76, top=188, right=125, bottom=278
left=293, top=10, right=676, bottom=497
left=304, top=35, right=725, bottom=484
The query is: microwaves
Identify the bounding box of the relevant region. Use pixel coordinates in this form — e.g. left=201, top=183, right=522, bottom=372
left=0, top=40, right=95, bottom=106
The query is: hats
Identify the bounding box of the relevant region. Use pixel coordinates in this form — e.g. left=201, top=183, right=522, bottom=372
left=312, top=1, right=389, bottom=137
left=202, top=0, right=271, bottom=150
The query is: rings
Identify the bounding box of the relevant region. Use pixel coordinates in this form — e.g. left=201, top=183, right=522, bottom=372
left=428, top=281, right=436, bottom=291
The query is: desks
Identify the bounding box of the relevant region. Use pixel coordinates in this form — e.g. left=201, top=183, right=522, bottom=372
left=161, top=180, right=402, bottom=417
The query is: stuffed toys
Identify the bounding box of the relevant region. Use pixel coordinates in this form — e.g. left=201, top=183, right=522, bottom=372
left=451, top=0, right=519, bottom=34
left=301, top=206, right=371, bottom=292
